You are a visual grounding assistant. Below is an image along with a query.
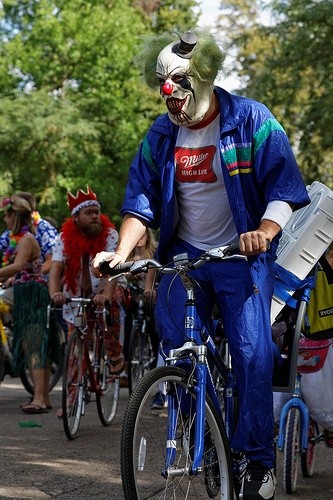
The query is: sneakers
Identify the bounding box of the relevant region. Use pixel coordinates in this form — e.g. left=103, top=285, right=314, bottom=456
left=237, top=461, right=277, bottom=499
left=181, top=414, right=190, bottom=457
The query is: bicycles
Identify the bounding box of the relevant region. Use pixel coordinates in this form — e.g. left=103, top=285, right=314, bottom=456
left=0, top=251, right=327, bottom=494
left=100, top=238, right=279, bottom=500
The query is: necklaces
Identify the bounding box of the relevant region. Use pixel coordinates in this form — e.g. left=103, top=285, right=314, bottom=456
left=1, top=211, right=41, bottom=269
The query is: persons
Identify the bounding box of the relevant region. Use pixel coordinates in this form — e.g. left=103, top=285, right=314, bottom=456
left=102, top=226, right=168, bottom=409
left=48, top=186, right=126, bottom=418
left=265, top=243, right=332, bottom=448
left=0, top=192, right=60, bottom=413
left=91, top=38, right=311, bottom=500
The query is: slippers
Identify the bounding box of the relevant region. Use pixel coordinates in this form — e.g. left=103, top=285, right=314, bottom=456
left=21, top=403, right=52, bottom=413
left=57, top=403, right=75, bottom=418
left=109, top=351, right=125, bottom=374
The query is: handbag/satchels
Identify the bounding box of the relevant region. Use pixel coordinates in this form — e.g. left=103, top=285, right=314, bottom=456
left=299, top=255, right=332, bottom=340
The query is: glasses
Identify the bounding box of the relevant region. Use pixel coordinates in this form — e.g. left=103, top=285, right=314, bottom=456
left=78, top=208, right=101, bottom=216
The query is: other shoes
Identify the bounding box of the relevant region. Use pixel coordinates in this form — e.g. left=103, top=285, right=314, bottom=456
left=150, top=393, right=166, bottom=409
left=324, top=428, right=333, bottom=447
left=118, top=375, right=129, bottom=388
